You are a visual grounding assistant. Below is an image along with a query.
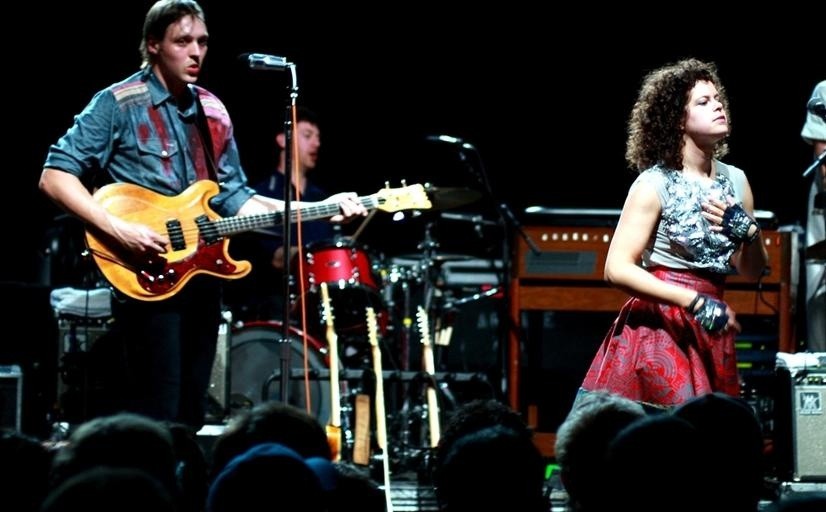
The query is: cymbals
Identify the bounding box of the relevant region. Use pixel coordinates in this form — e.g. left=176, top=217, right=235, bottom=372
left=427, top=186, right=480, bottom=210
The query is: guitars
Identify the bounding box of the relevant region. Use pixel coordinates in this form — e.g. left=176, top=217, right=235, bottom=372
left=85, top=181, right=436, bottom=304
left=366, top=306, right=394, bottom=512
left=415, top=306, right=444, bottom=471
left=323, top=281, right=344, bottom=461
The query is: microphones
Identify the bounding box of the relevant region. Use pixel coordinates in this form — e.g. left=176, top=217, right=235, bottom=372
left=425, top=134, right=473, bottom=150
left=237, top=53, right=293, bottom=72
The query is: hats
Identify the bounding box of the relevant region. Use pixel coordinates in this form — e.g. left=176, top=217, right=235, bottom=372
left=672, top=390, right=765, bottom=460
left=207, top=441, right=338, bottom=511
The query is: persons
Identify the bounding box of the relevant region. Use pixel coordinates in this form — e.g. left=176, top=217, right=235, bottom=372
left=568, top=59, right=768, bottom=418
left=37, top=0, right=369, bottom=432
left=230, top=109, right=350, bottom=320
left=801, top=80, right=826, bottom=351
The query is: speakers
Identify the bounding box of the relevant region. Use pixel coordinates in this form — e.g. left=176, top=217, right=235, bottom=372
left=772, top=351, right=826, bottom=483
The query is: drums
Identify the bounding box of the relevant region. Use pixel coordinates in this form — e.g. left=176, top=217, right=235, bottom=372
left=290, top=240, right=382, bottom=336
left=229, top=320, right=350, bottom=431
